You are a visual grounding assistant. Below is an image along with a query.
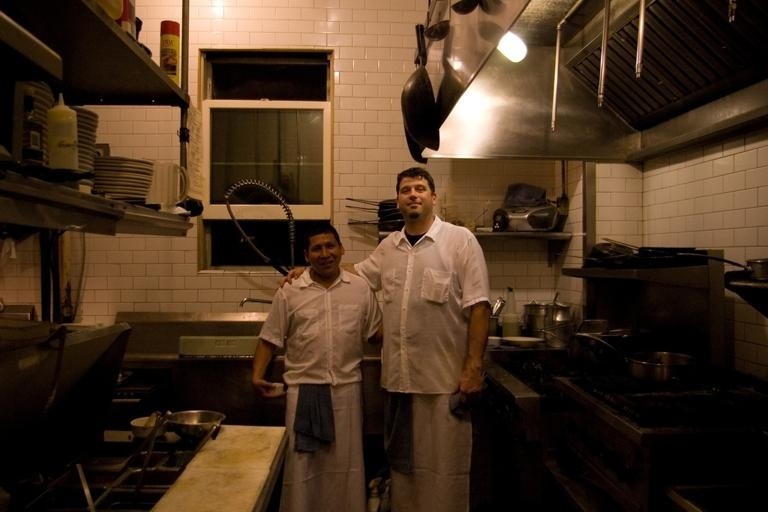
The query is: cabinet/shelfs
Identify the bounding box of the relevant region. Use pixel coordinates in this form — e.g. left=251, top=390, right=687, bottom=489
left=0, top=0, right=193, bottom=238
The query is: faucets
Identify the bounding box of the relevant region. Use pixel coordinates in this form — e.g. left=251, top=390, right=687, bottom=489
left=240, top=298, right=273, bottom=308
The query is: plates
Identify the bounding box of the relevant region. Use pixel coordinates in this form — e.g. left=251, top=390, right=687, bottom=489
left=502, top=336, right=542, bottom=348
left=27, top=79, right=154, bottom=205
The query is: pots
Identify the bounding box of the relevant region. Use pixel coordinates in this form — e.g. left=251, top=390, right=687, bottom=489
left=676, top=251, right=767, bottom=283
left=520, top=291, right=574, bottom=351
left=552, top=238, right=694, bottom=268
left=345, top=197, right=404, bottom=231
left=400, top=23, right=443, bottom=165
left=576, top=332, right=700, bottom=389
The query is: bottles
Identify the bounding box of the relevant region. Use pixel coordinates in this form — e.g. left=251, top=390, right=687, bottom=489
left=501, top=291, right=519, bottom=337
left=47, top=91, right=79, bottom=170
left=160, top=20, right=180, bottom=86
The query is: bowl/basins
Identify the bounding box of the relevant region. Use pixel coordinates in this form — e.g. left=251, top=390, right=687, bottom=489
left=487, top=337, right=500, bottom=348
left=130, top=417, right=165, bottom=438
left=168, top=409, right=225, bottom=438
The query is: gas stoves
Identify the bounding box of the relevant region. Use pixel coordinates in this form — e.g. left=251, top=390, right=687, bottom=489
left=577, top=362, right=767, bottom=423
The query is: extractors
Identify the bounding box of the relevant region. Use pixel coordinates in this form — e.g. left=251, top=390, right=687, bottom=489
left=559, top=0, right=767, bottom=132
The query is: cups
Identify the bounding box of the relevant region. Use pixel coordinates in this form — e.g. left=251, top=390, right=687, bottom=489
left=147, top=163, right=190, bottom=212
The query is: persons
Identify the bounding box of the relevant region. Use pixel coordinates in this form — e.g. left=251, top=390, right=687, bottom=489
left=276, top=167, right=491, bottom=510
left=250, top=222, right=384, bottom=510
